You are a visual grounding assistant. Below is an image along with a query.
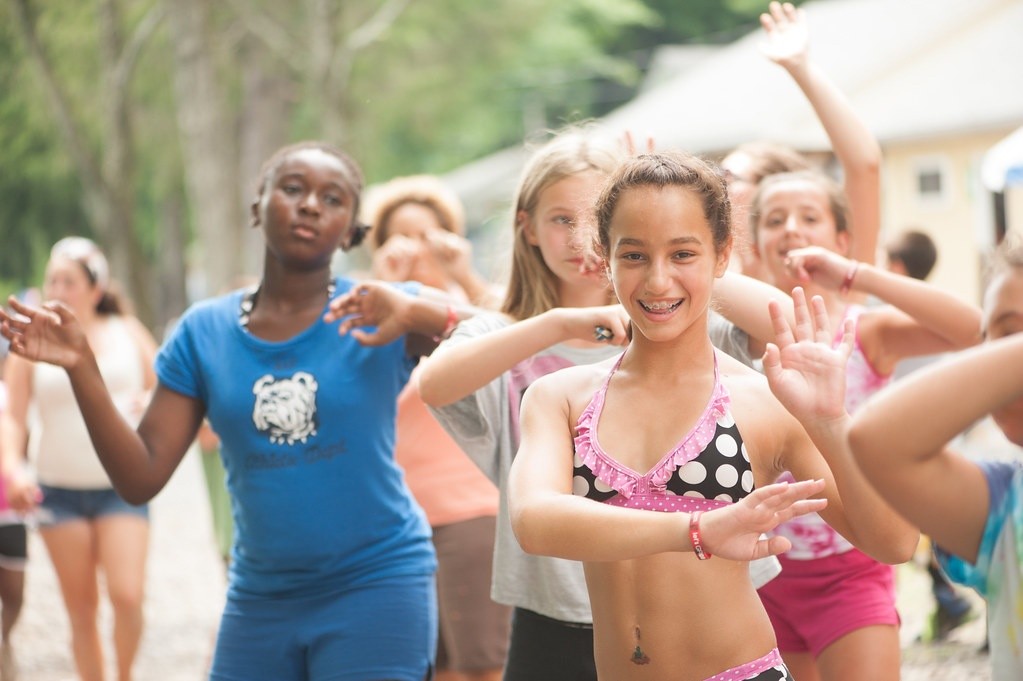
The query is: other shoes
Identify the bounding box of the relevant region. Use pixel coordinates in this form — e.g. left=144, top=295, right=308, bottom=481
left=921, top=591, right=982, bottom=645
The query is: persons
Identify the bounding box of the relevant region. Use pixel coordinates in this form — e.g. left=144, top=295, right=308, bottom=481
left=0, top=2, right=1023, bottom=681
left=501, top=147, right=923, bottom=681
left=0, top=135, right=483, bottom=680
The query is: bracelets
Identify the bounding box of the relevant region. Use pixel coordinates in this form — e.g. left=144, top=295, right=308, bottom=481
left=431, top=302, right=463, bottom=343
left=842, top=259, right=860, bottom=294
left=688, top=507, right=716, bottom=564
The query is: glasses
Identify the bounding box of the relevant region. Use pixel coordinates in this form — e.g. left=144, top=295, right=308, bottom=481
left=722, top=170, right=757, bottom=185
